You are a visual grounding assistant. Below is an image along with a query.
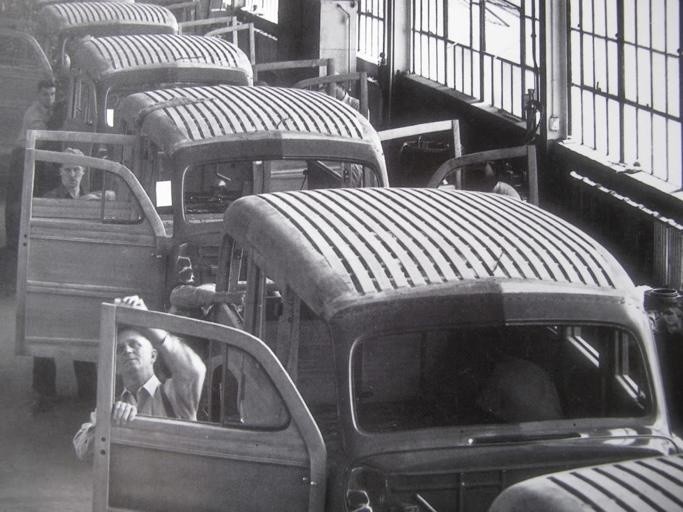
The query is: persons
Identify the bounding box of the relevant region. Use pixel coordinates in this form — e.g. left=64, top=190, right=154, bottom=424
left=28, top=144, right=97, bottom=416
left=3, top=80, right=57, bottom=250
left=71, top=294, right=208, bottom=459
left=472, top=162, right=521, bottom=199
left=322, top=82, right=370, bottom=123
left=471, top=334, right=563, bottom=423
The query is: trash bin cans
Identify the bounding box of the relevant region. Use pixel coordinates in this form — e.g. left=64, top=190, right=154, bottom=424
left=398, top=140, right=449, bottom=186
